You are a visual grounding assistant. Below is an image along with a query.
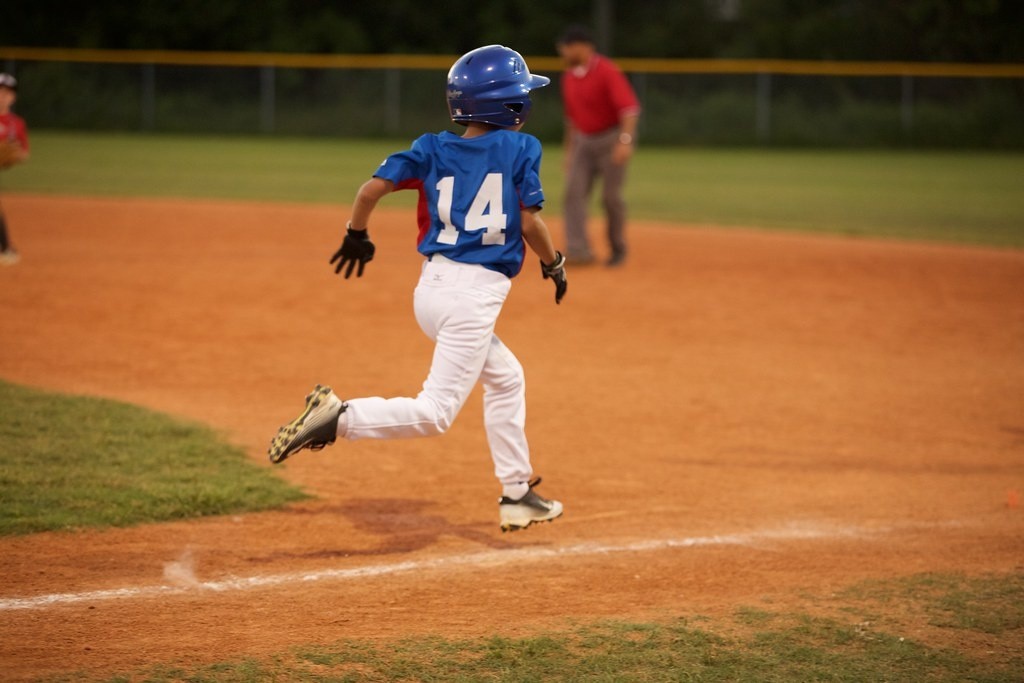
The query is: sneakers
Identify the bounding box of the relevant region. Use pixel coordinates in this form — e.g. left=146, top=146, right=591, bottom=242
left=268, top=384, right=348, bottom=464
left=498, top=477, right=564, bottom=532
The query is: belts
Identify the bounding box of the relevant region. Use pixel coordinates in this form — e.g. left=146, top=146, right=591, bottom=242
left=428, top=255, right=432, bottom=263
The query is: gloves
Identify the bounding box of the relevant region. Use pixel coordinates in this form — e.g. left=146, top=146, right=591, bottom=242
left=330, top=224, right=375, bottom=279
left=540, top=250, right=568, bottom=305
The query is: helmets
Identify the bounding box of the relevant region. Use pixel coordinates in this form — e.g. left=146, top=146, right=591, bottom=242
left=446, top=44, right=551, bottom=126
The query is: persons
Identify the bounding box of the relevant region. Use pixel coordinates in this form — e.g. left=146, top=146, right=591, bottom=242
left=267, top=45, right=562, bottom=533
left=562, top=38, right=638, bottom=268
left=0, top=68, right=31, bottom=255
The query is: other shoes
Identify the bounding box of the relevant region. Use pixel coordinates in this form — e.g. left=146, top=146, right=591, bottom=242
left=0, top=250, right=20, bottom=264
left=607, top=249, right=627, bottom=267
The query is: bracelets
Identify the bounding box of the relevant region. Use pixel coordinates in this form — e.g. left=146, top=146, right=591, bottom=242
left=540, top=250, right=566, bottom=270
left=619, top=131, right=632, bottom=144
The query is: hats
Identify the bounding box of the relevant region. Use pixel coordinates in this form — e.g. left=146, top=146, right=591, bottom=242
left=0, top=74, right=18, bottom=92
